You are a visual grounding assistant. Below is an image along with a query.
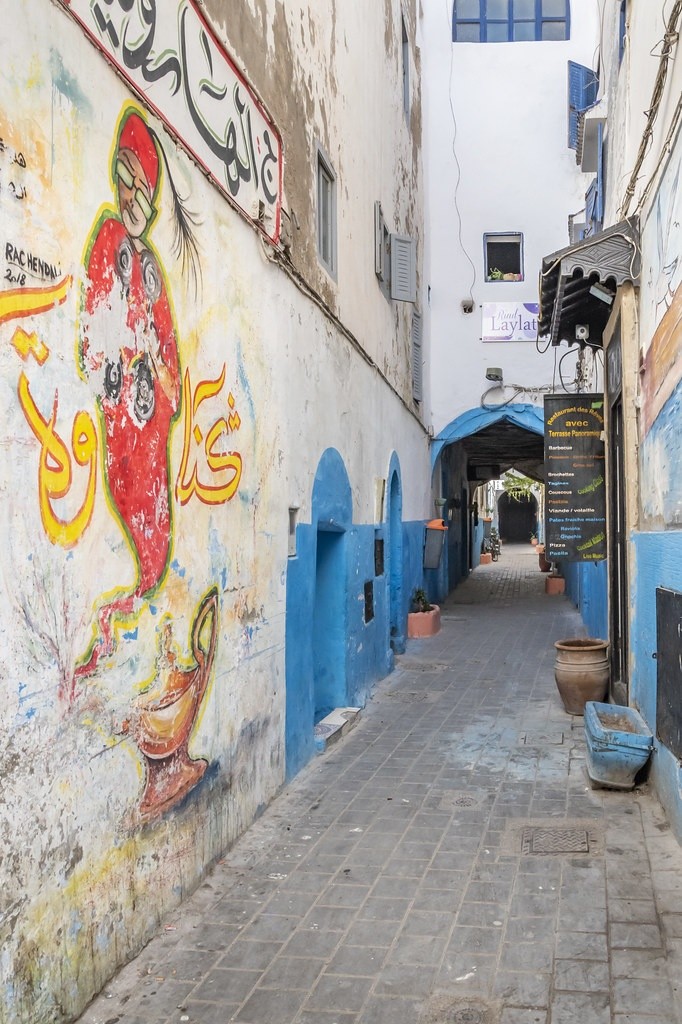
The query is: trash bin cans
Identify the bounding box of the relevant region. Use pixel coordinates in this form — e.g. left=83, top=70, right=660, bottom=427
left=423, top=518, right=448, bottom=569
left=483, top=518, right=491, bottom=538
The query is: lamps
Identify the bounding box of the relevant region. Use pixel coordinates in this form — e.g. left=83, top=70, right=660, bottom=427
left=589, top=282, right=614, bottom=305
left=486, top=368, right=503, bottom=382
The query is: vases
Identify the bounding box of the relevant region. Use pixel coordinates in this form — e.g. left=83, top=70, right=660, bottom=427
left=553, top=637, right=610, bottom=715
left=584, top=700, right=653, bottom=788
left=503, top=273, right=521, bottom=281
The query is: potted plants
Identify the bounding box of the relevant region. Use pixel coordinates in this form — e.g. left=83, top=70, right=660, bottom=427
left=409, top=588, right=441, bottom=636
left=490, top=267, right=504, bottom=280
left=530, top=531, right=538, bottom=544
left=480, top=546, right=492, bottom=564
left=543, top=546, right=566, bottom=595
